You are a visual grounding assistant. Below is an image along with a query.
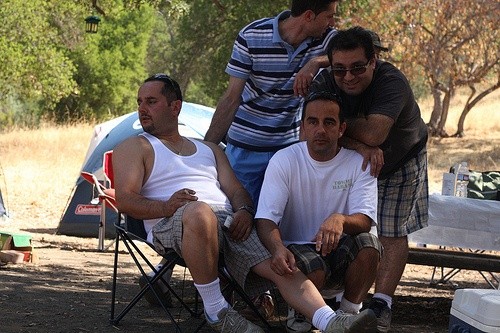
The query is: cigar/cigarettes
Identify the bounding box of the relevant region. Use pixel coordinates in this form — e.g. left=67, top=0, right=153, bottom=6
left=183, top=189, right=190, bottom=194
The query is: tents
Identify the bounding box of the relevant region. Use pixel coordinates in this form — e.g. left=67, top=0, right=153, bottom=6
left=57, top=101, right=216, bottom=237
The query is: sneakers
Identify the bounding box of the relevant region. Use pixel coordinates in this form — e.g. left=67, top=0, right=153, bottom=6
left=366, top=298, right=392, bottom=333
left=285, top=304, right=312, bottom=333
left=204, top=303, right=264, bottom=333
left=321, top=308, right=377, bottom=333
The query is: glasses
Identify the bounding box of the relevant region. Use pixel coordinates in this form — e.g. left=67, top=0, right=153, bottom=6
left=331, top=59, right=371, bottom=77
left=150, top=74, right=175, bottom=87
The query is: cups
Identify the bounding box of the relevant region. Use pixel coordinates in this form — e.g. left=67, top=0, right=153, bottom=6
left=442, top=172, right=455, bottom=196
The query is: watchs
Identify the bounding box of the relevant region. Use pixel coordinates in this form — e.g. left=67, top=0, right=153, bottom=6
left=239, top=204, right=255, bottom=215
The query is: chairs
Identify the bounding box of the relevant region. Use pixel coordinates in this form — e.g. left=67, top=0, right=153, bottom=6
left=80, top=149, right=263, bottom=333
left=428, top=166, right=500, bottom=289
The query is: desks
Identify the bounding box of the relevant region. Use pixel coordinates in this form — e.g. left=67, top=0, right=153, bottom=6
left=407, top=193, right=500, bottom=252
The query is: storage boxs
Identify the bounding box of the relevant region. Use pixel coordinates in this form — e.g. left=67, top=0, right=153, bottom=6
left=449, top=289, right=500, bottom=333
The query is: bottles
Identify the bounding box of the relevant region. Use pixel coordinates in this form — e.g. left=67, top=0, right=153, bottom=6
left=455, top=161, right=469, bottom=198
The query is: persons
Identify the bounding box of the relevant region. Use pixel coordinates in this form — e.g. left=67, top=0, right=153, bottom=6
left=253, top=89, right=379, bottom=333
left=327, top=26, right=430, bottom=333
left=114, top=74, right=379, bottom=333
left=202, top=0, right=347, bottom=230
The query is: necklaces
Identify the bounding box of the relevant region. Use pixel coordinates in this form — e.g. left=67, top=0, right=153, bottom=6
left=160, top=134, right=183, bottom=155
